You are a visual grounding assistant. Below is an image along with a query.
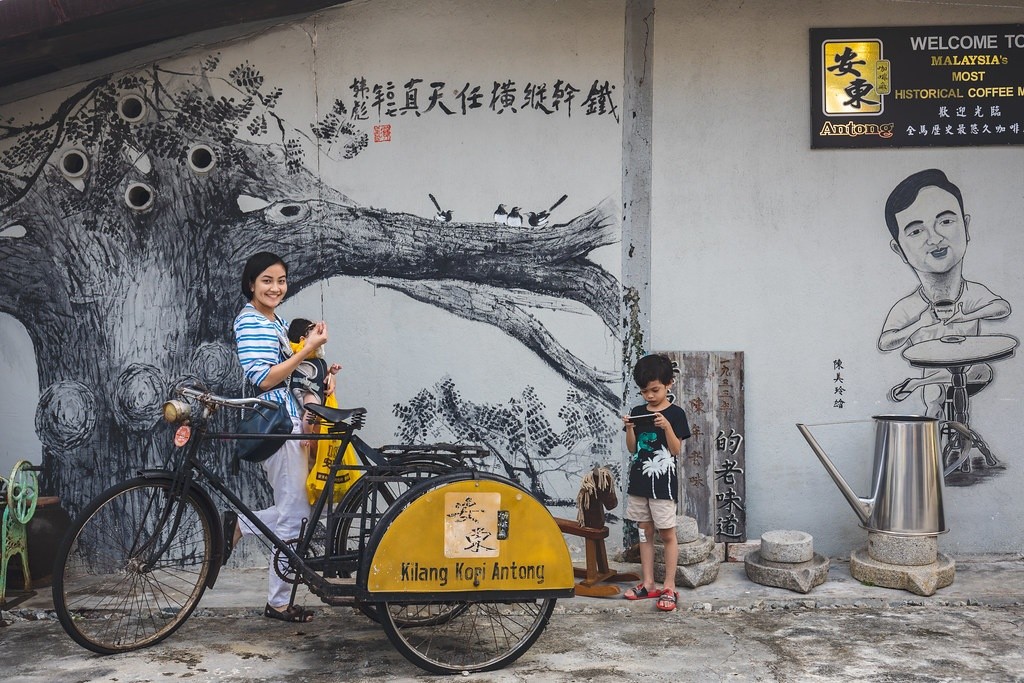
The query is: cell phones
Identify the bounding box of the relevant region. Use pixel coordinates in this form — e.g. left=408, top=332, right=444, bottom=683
left=628, top=414, right=657, bottom=427
left=326, top=372, right=332, bottom=392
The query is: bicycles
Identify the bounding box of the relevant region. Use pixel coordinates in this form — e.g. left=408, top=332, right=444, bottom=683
left=50, top=386, right=576, bottom=674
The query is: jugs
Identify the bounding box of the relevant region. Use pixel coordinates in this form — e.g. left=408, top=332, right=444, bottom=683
left=795, top=415, right=972, bottom=538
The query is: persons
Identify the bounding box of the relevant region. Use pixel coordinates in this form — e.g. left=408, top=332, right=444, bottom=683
left=221, top=251, right=314, bottom=623
left=622, top=354, right=692, bottom=610
left=287, top=316, right=343, bottom=446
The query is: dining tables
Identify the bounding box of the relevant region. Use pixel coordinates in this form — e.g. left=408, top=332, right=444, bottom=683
left=900, top=333, right=1020, bottom=471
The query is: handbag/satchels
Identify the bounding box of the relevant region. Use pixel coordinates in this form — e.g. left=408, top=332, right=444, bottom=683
left=307, top=395, right=365, bottom=506
left=234, top=401, right=294, bottom=463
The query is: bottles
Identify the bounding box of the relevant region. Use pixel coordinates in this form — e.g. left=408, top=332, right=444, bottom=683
left=21, top=496, right=74, bottom=578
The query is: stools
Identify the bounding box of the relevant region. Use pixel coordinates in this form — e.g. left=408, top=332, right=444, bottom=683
left=939, top=397, right=957, bottom=442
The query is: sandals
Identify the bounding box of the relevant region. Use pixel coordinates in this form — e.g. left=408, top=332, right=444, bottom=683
left=221, top=510, right=238, bottom=565
left=264, top=603, right=313, bottom=623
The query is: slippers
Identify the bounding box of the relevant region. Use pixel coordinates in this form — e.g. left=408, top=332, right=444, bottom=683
left=657, top=587, right=678, bottom=610
left=624, top=582, right=662, bottom=600
